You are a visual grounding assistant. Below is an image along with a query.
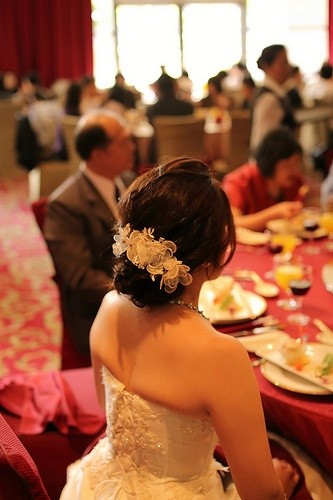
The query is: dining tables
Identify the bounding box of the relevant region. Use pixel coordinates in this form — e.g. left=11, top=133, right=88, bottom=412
left=195, top=209, right=333, bottom=480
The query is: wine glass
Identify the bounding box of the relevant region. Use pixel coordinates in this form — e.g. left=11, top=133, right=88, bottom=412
left=286, top=265, right=312, bottom=325
left=301, top=207, right=321, bottom=255
left=274, top=254, right=303, bottom=309
left=263, top=230, right=284, bottom=278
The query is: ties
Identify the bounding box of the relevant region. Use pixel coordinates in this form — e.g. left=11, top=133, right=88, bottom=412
left=114, top=185, right=119, bottom=204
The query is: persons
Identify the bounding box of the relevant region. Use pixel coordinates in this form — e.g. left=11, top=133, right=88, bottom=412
left=42, top=108, right=137, bottom=355
left=221, top=129, right=312, bottom=233
left=59, top=156, right=300, bottom=500
left=0, top=45, right=333, bottom=182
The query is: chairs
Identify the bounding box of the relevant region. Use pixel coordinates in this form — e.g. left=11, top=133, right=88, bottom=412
left=0, top=90, right=333, bottom=500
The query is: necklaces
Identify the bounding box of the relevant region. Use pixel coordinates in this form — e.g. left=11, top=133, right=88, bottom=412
left=168, top=299, right=211, bottom=322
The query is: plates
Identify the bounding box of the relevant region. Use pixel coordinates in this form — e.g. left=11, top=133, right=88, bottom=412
left=198, top=291, right=267, bottom=325
left=261, top=344, right=333, bottom=395
